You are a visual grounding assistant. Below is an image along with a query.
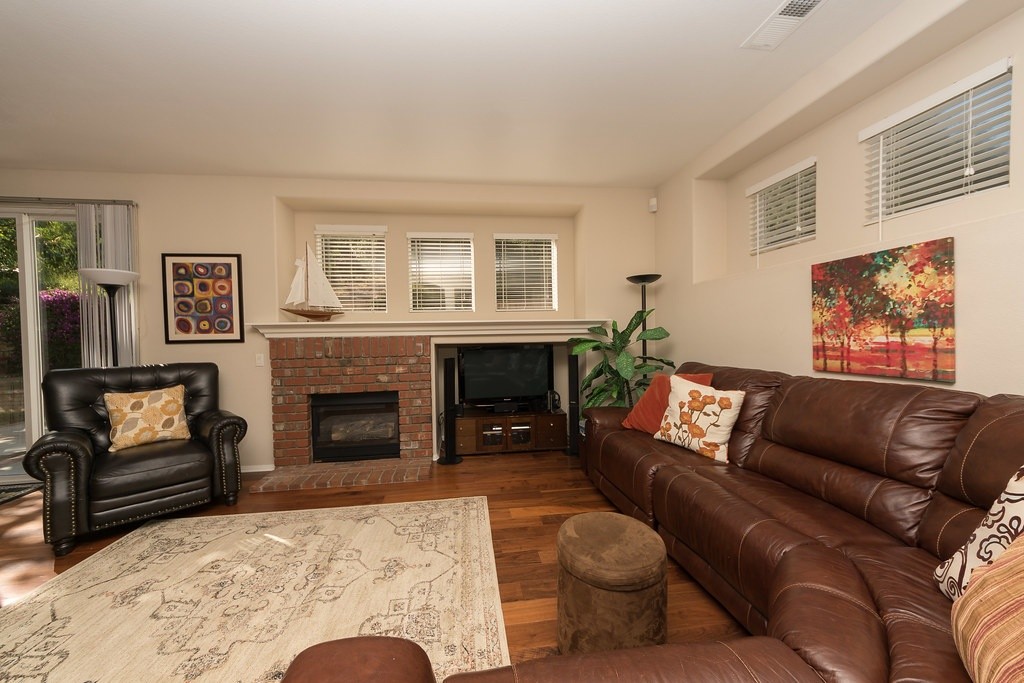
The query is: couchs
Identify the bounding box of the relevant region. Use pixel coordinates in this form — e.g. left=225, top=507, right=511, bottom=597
left=22, top=364, right=248, bottom=558
left=280, top=361, right=1024, bottom=683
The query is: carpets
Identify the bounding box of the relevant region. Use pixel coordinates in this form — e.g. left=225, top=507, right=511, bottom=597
left=0, top=482, right=44, bottom=505
left=0, top=496, right=511, bottom=683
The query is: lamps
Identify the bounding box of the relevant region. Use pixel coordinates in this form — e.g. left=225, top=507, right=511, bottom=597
left=79, top=268, right=140, bottom=367
left=626, top=274, right=662, bottom=392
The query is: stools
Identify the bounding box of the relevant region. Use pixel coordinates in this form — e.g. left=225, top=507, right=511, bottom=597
left=557, top=512, right=669, bottom=654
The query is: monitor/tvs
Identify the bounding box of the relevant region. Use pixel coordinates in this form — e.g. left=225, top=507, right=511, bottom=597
left=457, top=343, right=554, bottom=414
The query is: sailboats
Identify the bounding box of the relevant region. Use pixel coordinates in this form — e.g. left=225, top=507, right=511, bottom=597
left=276, top=240, right=346, bottom=322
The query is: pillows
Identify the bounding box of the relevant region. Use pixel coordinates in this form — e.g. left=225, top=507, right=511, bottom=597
left=950, top=535, right=1024, bottom=683
left=934, top=465, right=1024, bottom=601
left=103, top=385, right=191, bottom=453
left=621, top=372, right=713, bottom=434
left=653, top=375, right=746, bottom=463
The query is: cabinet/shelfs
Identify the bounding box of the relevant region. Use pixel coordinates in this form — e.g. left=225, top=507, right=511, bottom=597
left=456, top=409, right=569, bottom=456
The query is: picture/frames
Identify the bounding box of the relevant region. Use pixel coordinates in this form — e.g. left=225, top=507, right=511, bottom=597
left=161, top=252, right=247, bottom=345
